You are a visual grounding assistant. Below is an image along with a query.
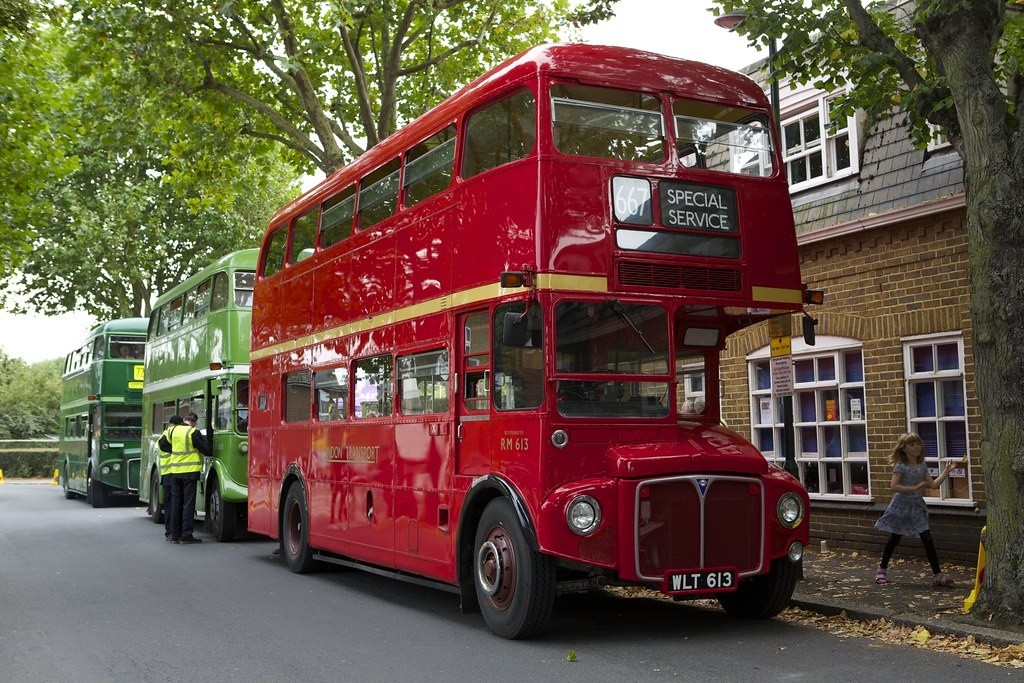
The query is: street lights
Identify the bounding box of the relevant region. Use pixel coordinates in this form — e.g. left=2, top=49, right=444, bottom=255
left=716, top=7, right=800, bottom=485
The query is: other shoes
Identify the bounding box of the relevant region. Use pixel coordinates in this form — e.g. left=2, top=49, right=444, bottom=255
left=165, top=532, right=202, bottom=545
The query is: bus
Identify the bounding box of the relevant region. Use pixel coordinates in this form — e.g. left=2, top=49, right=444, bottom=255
left=56, top=316, right=153, bottom=507
left=140, top=246, right=314, bottom=543
left=251, top=37, right=830, bottom=643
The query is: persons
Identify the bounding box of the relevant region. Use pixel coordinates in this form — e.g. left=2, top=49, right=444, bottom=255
left=167, top=413, right=210, bottom=543
left=218, top=414, right=248, bottom=433
left=117, top=346, right=134, bottom=360
left=153, top=416, right=183, bottom=538
left=875, top=432, right=958, bottom=584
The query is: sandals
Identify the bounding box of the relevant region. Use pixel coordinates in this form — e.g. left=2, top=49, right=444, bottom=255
left=875, top=567, right=887, bottom=584
left=933, top=573, right=955, bottom=586
